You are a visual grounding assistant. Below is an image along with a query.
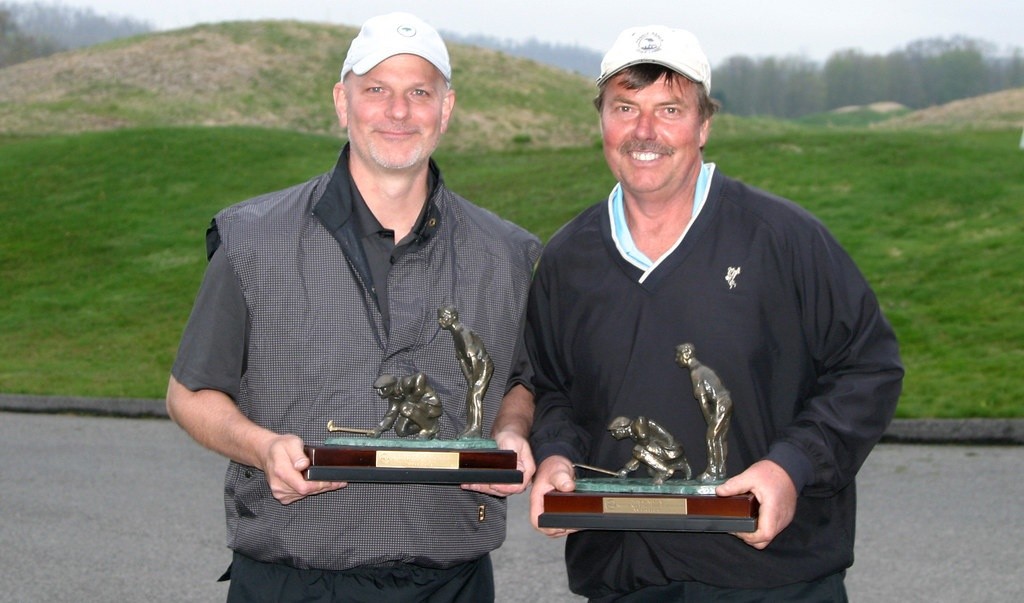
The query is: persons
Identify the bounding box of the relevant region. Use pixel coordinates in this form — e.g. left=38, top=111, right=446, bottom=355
left=675, top=343, right=734, bottom=482
left=527, top=25, right=904, bottom=603
left=165, top=13, right=544, bottom=603
left=607, top=415, right=692, bottom=484
left=367, top=372, right=444, bottom=439
left=437, top=306, right=494, bottom=440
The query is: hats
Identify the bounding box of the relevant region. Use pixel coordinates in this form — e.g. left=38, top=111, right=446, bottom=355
left=341, top=12, right=452, bottom=90
left=595, top=24, right=712, bottom=97
